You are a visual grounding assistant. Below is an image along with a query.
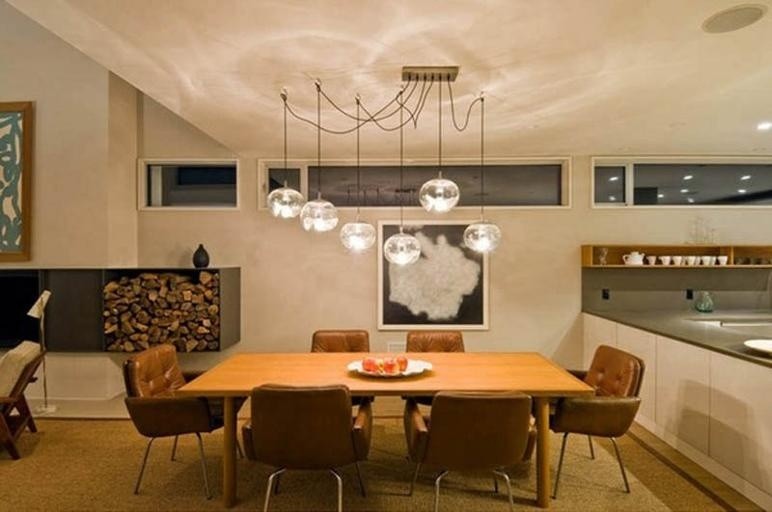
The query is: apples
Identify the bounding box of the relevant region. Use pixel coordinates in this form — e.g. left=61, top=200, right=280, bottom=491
left=362, top=355, right=408, bottom=373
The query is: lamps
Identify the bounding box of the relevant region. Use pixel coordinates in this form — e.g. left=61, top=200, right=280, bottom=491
left=265, top=66, right=502, bottom=268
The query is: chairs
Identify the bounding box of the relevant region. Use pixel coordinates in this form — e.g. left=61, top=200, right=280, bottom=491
left=528, top=343, right=647, bottom=498
left=242, top=379, right=376, bottom=512
left=401, top=385, right=537, bottom=511
left=404, top=328, right=466, bottom=352
left=310, top=328, right=372, bottom=354
left=1, top=340, right=48, bottom=460
left=121, top=343, right=244, bottom=500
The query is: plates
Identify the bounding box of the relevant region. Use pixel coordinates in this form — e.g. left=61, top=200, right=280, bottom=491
left=345, top=358, right=435, bottom=377
left=742, top=338, right=771, bottom=355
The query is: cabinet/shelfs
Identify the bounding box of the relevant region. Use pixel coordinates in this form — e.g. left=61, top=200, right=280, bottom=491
left=727, top=244, right=771, bottom=271
left=581, top=241, right=732, bottom=272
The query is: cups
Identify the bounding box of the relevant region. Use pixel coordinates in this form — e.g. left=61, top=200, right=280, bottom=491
left=733, top=254, right=771, bottom=266
left=647, top=254, right=730, bottom=267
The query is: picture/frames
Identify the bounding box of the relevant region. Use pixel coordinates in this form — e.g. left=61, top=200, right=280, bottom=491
left=377, top=218, right=490, bottom=333
left=1, top=100, right=33, bottom=262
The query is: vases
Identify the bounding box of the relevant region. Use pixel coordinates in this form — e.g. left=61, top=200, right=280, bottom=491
left=192, top=243, right=209, bottom=268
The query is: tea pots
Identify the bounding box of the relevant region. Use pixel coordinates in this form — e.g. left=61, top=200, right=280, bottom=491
left=620, top=251, right=646, bottom=266
left=694, top=288, right=720, bottom=311
